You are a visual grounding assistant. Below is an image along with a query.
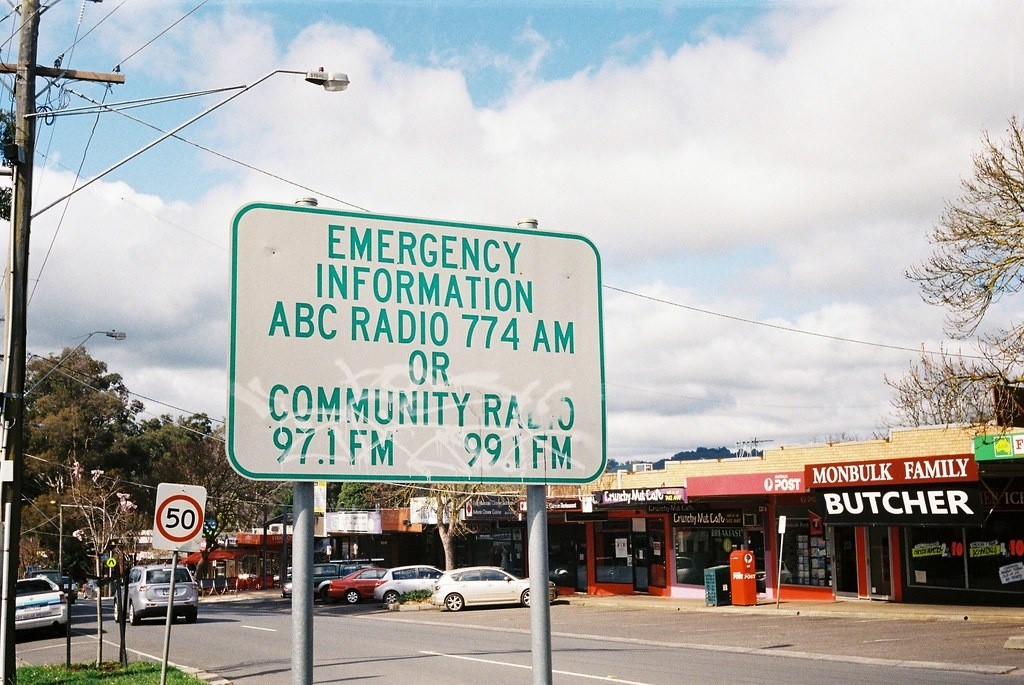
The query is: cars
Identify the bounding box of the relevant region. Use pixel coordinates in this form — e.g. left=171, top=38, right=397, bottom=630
left=676, top=556, right=702, bottom=585
left=272, top=562, right=374, bottom=604
left=62, top=575, right=79, bottom=604
left=428, top=566, right=559, bottom=613
left=328, top=568, right=390, bottom=604
left=15, top=576, right=69, bottom=642
left=372, top=564, right=445, bottom=604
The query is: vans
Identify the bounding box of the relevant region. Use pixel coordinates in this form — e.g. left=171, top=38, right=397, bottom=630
left=24, top=570, right=64, bottom=593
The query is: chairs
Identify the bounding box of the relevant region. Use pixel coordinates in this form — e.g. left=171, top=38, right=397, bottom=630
left=166, top=574, right=180, bottom=582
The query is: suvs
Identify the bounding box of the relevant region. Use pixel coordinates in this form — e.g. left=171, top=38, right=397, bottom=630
left=113, top=565, right=199, bottom=626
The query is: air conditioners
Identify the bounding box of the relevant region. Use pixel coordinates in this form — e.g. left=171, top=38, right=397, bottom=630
left=633, top=463, right=653, bottom=472
left=742, top=513, right=763, bottom=527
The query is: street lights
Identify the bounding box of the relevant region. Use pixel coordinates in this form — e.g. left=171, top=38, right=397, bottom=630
left=4, top=65, right=350, bottom=685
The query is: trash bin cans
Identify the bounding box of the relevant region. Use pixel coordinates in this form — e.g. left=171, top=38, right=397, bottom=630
left=704, top=565, right=730, bottom=607
left=85, top=584, right=93, bottom=599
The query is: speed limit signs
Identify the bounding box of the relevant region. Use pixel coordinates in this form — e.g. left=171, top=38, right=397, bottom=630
left=152, top=482, right=206, bottom=553
